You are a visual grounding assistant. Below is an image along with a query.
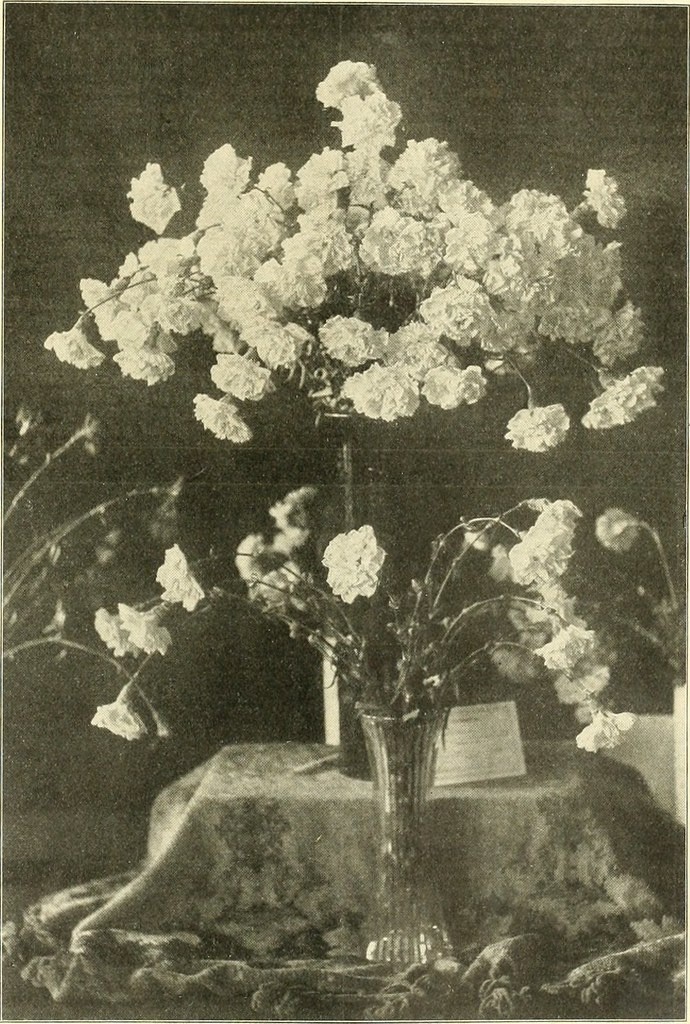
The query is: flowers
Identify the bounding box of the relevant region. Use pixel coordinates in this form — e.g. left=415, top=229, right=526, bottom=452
left=76, top=488, right=641, bottom=751
left=43, top=60, right=664, bottom=453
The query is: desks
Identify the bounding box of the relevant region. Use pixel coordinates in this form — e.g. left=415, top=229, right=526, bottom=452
left=3, top=746, right=685, bottom=1019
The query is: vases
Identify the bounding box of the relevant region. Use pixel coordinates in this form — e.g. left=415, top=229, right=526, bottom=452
left=318, top=442, right=390, bottom=748
left=360, top=707, right=455, bottom=965
